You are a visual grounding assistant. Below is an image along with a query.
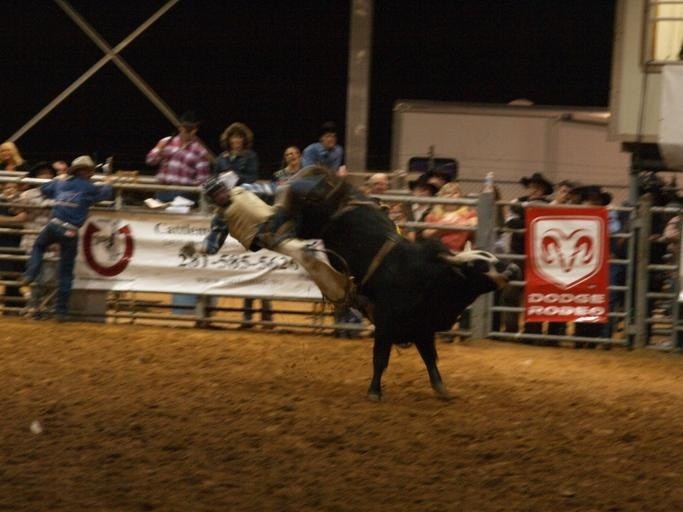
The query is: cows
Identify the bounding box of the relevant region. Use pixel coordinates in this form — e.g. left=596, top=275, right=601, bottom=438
left=246, top=163, right=523, bottom=402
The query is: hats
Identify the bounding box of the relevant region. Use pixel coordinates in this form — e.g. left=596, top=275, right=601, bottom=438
left=407, top=169, right=453, bottom=195
left=25, top=161, right=58, bottom=178
left=520, top=173, right=553, bottom=195
left=220, top=123, right=255, bottom=149
left=66, top=155, right=103, bottom=176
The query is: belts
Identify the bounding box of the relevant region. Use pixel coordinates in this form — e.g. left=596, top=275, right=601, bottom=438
left=51, top=217, right=77, bottom=231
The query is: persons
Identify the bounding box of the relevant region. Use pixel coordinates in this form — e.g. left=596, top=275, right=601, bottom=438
left=355, top=167, right=484, bottom=342
left=494, top=171, right=623, bottom=349
left=142, top=116, right=344, bottom=329
left=1, top=141, right=121, bottom=321
left=624, top=199, right=683, bottom=347
